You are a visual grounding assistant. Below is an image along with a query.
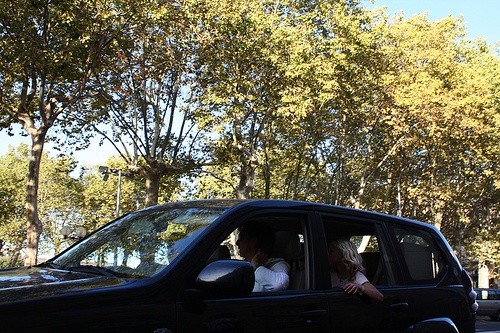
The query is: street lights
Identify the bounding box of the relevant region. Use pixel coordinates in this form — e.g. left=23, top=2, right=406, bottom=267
left=98, top=162, right=132, bottom=218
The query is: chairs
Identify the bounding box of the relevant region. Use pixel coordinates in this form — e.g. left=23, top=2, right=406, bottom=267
left=359, top=251, right=387, bottom=287
left=272, top=231, right=305, bottom=290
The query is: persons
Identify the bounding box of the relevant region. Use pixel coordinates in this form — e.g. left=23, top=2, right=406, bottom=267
left=142, top=231, right=190, bottom=274
left=329, top=237, right=386, bottom=303
left=486, top=278, right=499, bottom=299
left=235, top=217, right=291, bottom=293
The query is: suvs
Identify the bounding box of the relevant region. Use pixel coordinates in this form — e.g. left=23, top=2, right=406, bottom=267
left=0, top=198, right=478, bottom=333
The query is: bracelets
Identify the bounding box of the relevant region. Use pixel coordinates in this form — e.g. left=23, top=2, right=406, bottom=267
left=359, top=285, right=365, bottom=295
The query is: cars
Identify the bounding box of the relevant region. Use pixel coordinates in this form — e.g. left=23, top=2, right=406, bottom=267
left=472, top=288, right=500, bottom=322
